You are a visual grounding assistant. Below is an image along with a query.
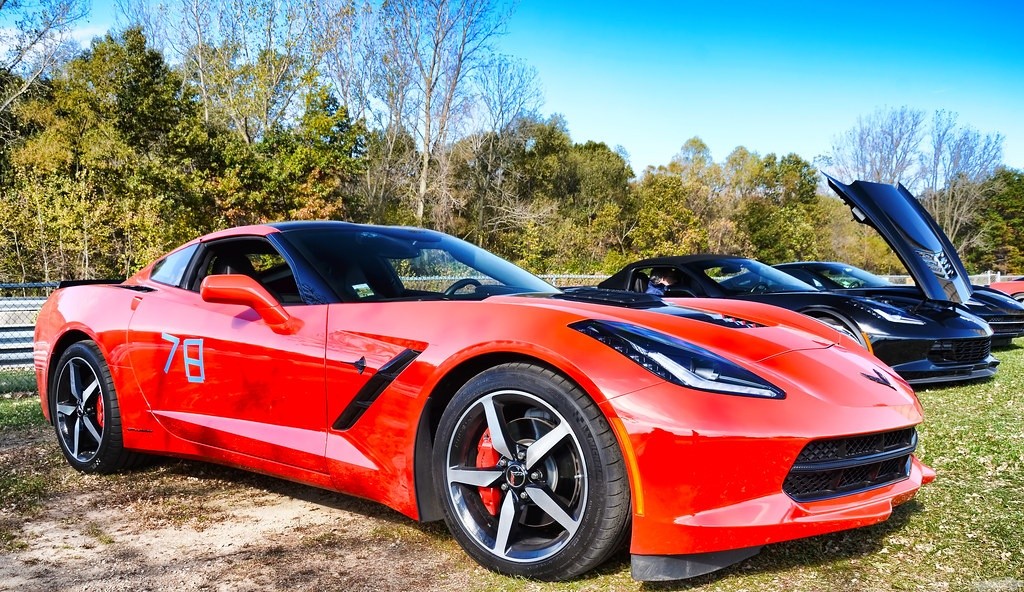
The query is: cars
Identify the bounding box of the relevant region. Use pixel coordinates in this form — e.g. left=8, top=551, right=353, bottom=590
left=983, top=275, right=1024, bottom=307
left=767, top=262, right=1024, bottom=350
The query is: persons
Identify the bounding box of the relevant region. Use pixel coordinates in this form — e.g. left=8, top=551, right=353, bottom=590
left=644, top=269, right=677, bottom=297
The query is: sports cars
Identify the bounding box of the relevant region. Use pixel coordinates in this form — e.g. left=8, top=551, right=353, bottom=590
left=558, top=165, right=1002, bottom=387
left=33, top=219, right=938, bottom=583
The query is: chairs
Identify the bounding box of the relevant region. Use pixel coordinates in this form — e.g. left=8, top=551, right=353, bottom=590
left=213, top=255, right=284, bottom=304
left=634, top=273, right=649, bottom=293
